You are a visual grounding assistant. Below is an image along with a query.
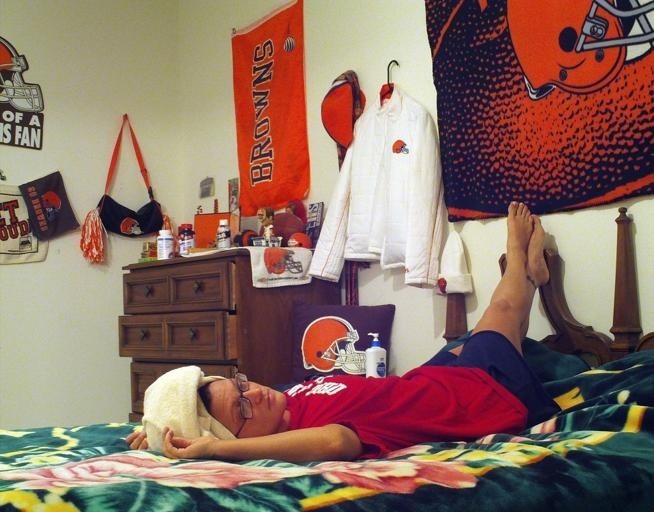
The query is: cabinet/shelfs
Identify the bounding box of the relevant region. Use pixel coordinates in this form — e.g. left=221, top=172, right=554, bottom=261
left=118, top=248, right=340, bottom=422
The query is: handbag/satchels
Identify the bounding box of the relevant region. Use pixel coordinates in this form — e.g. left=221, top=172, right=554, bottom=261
left=98, top=196, right=164, bottom=236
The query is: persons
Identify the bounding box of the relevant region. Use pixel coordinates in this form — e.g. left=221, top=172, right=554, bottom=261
left=124, top=202, right=552, bottom=462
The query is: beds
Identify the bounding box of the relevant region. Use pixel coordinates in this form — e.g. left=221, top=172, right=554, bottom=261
left=0, top=206, right=654, bottom=512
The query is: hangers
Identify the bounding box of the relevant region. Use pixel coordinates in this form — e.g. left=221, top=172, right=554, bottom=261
left=354, top=60, right=433, bottom=127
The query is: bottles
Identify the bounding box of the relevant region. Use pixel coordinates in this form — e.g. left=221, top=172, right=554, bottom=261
left=156, top=230, right=175, bottom=260
left=215, top=218, right=231, bottom=249
left=178, top=223, right=196, bottom=258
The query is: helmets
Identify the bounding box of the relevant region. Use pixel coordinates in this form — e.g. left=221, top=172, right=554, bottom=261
left=0, top=37, right=44, bottom=113
left=507, top=1, right=654, bottom=100
left=302, top=317, right=366, bottom=376
left=321, top=83, right=354, bottom=149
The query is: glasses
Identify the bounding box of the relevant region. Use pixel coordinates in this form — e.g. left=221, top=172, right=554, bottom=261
left=231, top=371, right=254, bottom=437
